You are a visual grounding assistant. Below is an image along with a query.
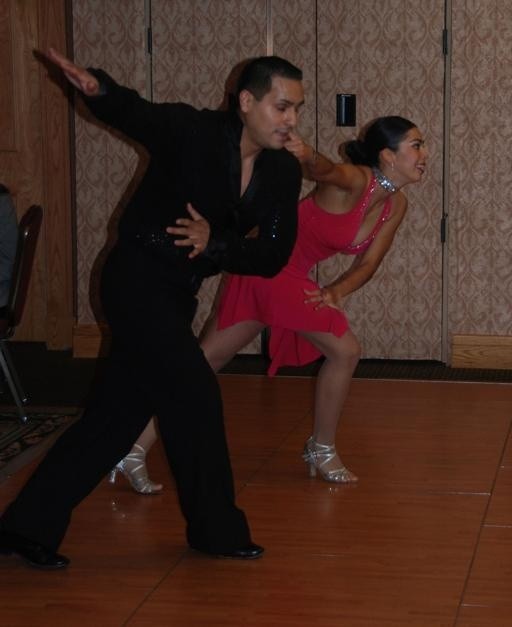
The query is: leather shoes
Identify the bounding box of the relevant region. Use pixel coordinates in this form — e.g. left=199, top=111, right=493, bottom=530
left=0, top=540, right=70, bottom=569
left=189, top=540, right=264, bottom=559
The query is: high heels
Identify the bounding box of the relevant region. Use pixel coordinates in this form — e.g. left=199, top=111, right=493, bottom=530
left=300, top=435, right=358, bottom=483
left=108, top=444, right=164, bottom=494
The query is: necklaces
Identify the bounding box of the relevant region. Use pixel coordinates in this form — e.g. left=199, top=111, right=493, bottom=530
left=369, top=163, right=400, bottom=197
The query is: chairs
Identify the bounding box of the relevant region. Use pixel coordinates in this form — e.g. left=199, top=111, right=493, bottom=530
left=0, top=206, right=43, bottom=426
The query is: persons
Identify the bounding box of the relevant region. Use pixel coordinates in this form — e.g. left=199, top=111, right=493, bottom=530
left=0, top=43, right=308, bottom=573
left=109, top=112, right=429, bottom=497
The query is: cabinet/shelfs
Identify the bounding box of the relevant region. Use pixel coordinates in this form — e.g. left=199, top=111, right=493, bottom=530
left=69, top=0, right=512, bottom=374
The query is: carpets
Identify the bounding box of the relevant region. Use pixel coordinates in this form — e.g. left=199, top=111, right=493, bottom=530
left=1, top=410, right=82, bottom=492
